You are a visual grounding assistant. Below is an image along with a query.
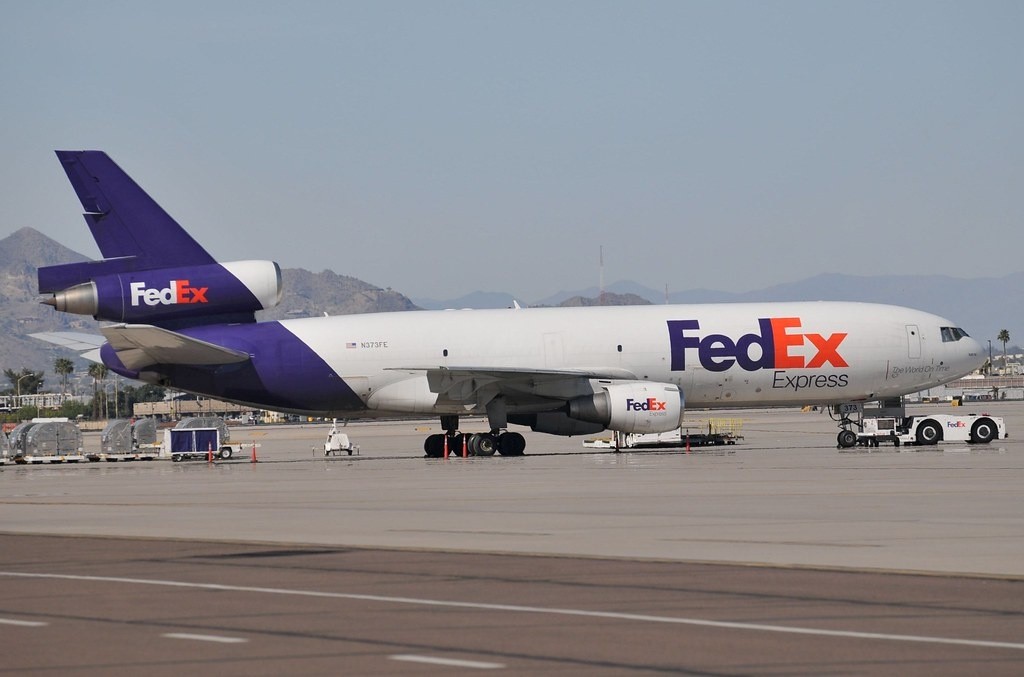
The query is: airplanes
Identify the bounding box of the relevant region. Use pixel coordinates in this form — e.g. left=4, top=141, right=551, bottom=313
left=38, top=149, right=988, bottom=458
